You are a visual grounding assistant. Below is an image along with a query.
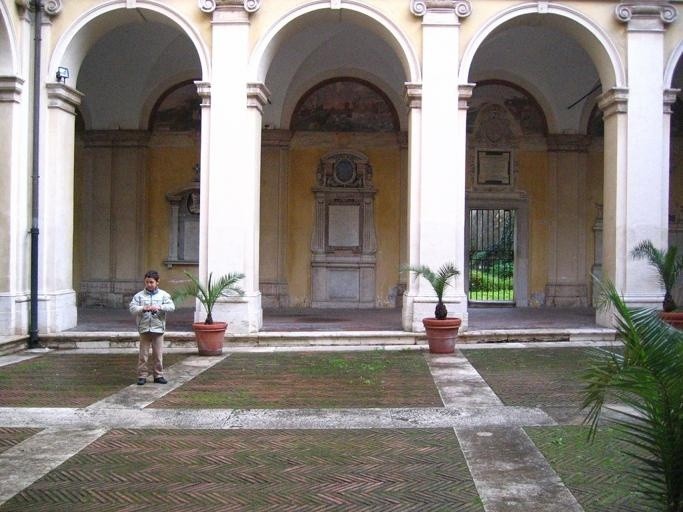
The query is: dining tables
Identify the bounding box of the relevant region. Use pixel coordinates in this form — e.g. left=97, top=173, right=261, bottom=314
left=630, top=241, right=683, bottom=330
left=404, top=258, right=462, bottom=355
left=170, top=268, right=246, bottom=355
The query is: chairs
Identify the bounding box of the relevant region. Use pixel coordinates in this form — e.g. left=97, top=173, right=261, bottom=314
left=153, top=377, right=166, bottom=384
left=136, top=378, right=146, bottom=385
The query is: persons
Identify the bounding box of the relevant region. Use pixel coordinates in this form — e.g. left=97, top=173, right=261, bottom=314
left=129, top=270, right=175, bottom=385
